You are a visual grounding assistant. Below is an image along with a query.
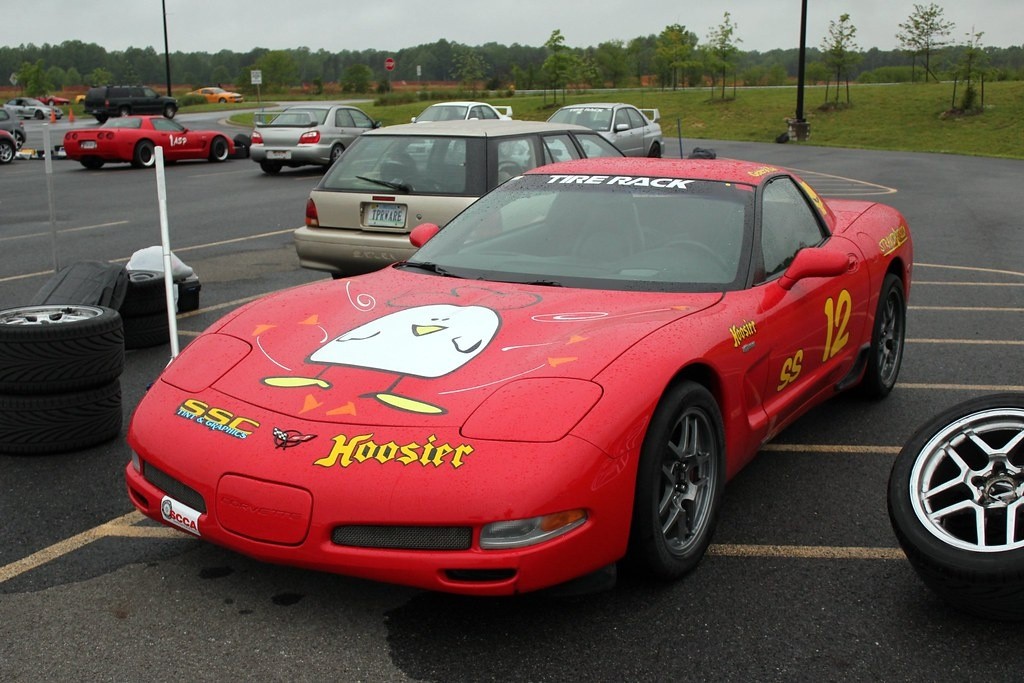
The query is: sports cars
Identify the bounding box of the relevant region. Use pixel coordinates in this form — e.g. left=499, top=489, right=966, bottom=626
left=62, top=114, right=235, bottom=168
left=122, top=153, right=916, bottom=604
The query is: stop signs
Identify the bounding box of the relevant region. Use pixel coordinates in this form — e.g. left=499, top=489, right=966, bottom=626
left=385, top=57, right=396, bottom=71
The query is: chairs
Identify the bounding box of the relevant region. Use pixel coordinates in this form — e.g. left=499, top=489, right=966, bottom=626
left=533, top=189, right=646, bottom=271
left=676, top=194, right=781, bottom=281
left=381, top=151, right=416, bottom=181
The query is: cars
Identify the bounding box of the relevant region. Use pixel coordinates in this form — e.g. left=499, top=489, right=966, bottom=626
left=400, top=102, right=517, bottom=162
left=0, top=109, right=27, bottom=150
left=184, top=86, right=244, bottom=104
left=0, top=127, right=18, bottom=165
left=511, top=102, right=665, bottom=175
left=35, top=94, right=71, bottom=106
left=248, top=102, right=385, bottom=174
left=289, top=117, right=644, bottom=283
left=3, top=98, right=63, bottom=119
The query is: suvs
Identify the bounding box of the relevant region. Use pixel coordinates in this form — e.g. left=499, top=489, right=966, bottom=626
left=84, top=82, right=178, bottom=124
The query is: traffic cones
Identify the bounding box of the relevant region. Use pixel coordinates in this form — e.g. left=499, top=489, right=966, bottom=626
left=68, top=108, right=75, bottom=123
left=49, top=107, right=57, bottom=123
